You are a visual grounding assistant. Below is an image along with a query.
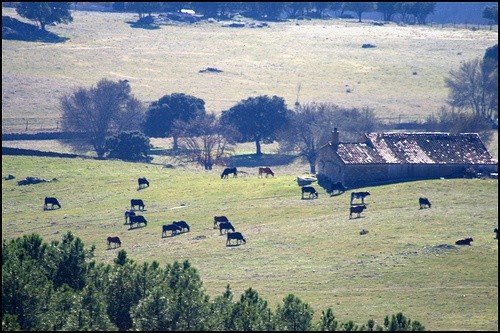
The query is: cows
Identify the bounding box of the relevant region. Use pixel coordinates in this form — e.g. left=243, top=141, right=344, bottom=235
left=106, top=237, right=121, bottom=248
left=218, top=222, right=235, bottom=236
left=493, top=228, right=498, bottom=239
left=124, top=210, right=148, bottom=228
left=226, top=231, right=246, bottom=245
left=44, top=196, right=62, bottom=209
left=301, top=187, right=318, bottom=199
left=455, top=238, right=473, bottom=246
left=130, top=198, right=145, bottom=211
left=349, top=205, right=367, bottom=217
left=330, top=182, right=348, bottom=196
left=220, top=167, right=237, bottom=179
left=161, top=220, right=191, bottom=237
left=258, top=167, right=274, bottom=178
left=351, top=192, right=370, bottom=204
left=137, top=177, right=150, bottom=189
left=418, top=197, right=431, bottom=209
left=214, top=216, right=229, bottom=229
